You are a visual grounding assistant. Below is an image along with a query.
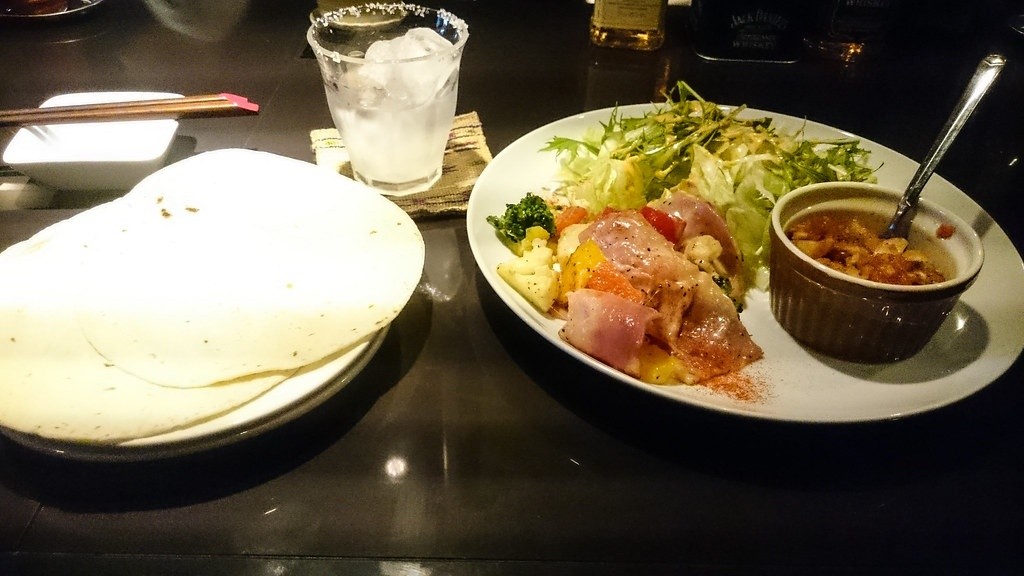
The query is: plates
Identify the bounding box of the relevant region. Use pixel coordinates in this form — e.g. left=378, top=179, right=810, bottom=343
left=0, top=226, right=392, bottom=460
left=467, top=102, right=1024, bottom=420
left=0, top=0, right=102, bottom=21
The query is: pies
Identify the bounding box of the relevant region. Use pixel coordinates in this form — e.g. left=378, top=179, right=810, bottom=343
left=0, top=148, right=425, bottom=439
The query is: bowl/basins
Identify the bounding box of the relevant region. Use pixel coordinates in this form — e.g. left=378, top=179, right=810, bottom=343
left=770, top=181, right=985, bottom=362
left=3, top=90, right=186, bottom=190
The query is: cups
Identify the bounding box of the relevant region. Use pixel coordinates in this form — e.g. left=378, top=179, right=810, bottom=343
left=589, top=0, right=666, bottom=51
left=308, top=4, right=468, bottom=195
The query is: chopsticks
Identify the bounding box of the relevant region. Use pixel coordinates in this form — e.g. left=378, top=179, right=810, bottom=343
left=0, top=93, right=259, bottom=128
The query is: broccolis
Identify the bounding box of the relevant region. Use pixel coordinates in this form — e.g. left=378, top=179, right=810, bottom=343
left=485, top=192, right=563, bottom=312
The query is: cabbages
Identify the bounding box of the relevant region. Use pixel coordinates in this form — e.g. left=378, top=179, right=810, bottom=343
left=556, top=126, right=878, bottom=290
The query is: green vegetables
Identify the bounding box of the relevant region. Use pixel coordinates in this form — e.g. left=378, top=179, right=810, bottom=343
left=536, top=80, right=883, bottom=193
